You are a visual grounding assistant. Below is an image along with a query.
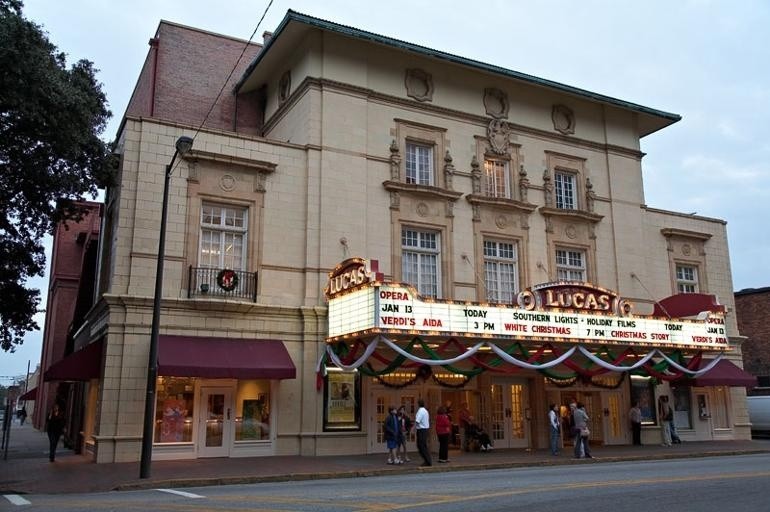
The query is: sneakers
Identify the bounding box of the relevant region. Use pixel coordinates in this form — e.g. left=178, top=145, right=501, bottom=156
left=387, top=457, right=411, bottom=463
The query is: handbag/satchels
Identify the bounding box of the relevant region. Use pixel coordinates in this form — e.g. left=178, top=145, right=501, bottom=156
left=580, top=426, right=591, bottom=438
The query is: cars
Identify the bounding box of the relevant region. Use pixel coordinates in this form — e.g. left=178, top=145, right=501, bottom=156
left=159, top=410, right=270, bottom=442
left=0, top=410, right=5, bottom=420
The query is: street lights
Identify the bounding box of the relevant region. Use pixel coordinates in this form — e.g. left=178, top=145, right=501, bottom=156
left=137, top=133, right=199, bottom=478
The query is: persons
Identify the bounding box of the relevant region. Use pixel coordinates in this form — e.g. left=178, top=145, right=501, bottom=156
left=383, top=398, right=494, bottom=467
left=548, top=403, right=561, bottom=456
left=659, top=394, right=681, bottom=447
left=573, top=402, right=595, bottom=458
left=20, top=406, right=27, bottom=425
left=45, top=402, right=64, bottom=462
left=628, top=401, right=644, bottom=446
